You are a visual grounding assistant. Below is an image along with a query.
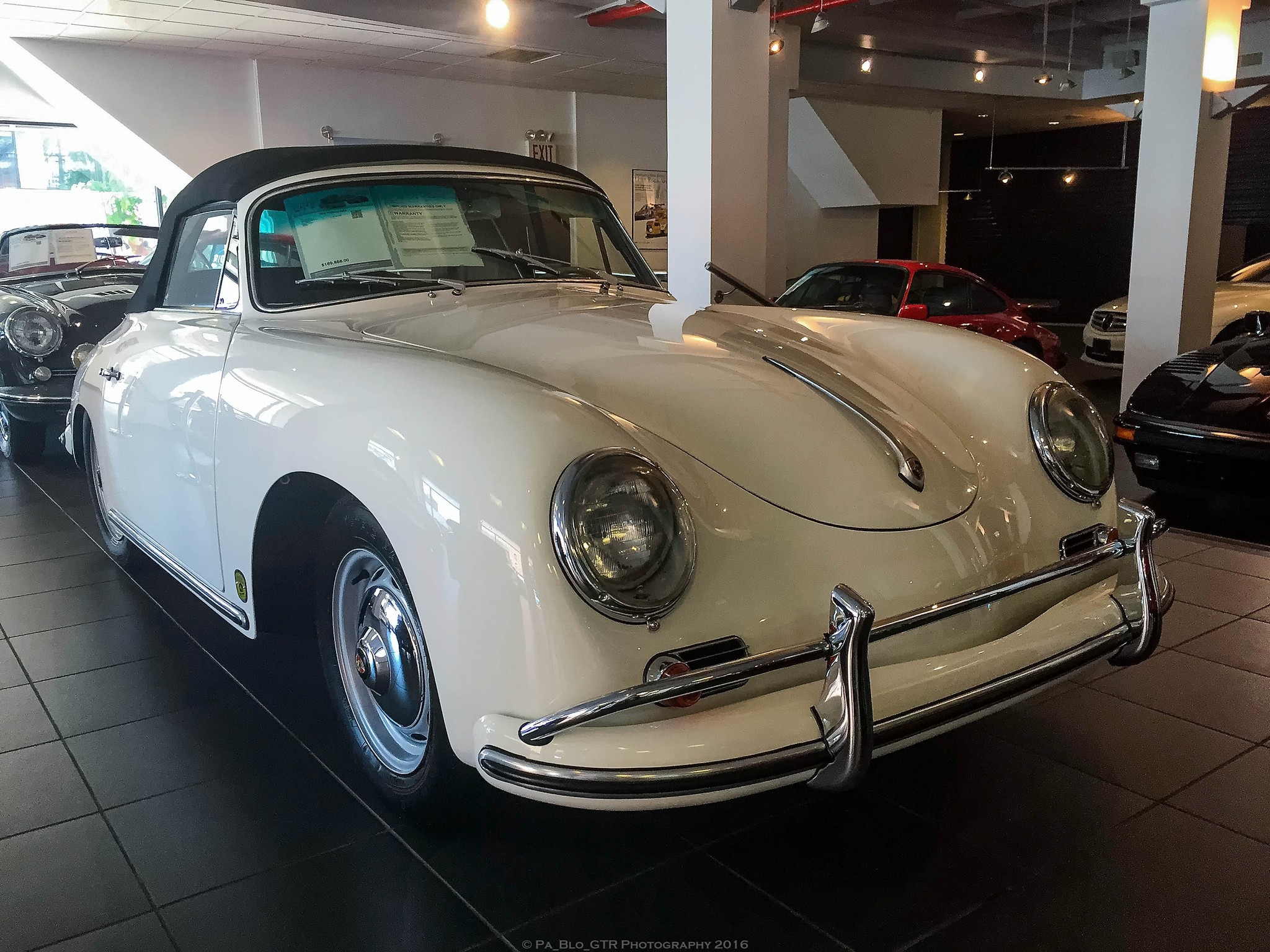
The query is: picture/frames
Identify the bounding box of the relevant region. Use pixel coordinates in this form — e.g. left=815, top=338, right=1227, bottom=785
left=631, top=169, right=668, bottom=251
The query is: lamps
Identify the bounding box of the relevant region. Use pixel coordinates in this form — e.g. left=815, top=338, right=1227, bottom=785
left=1062, top=168, right=1074, bottom=183
left=547, top=132, right=555, bottom=142
left=1104, top=98, right=1144, bottom=121
left=1032, top=5, right=1053, bottom=85
left=812, top=0, right=830, bottom=35
left=1112, top=0, right=1139, bottom=80
left=525, top=129, right=535, bottom=140
left=536, top=129, right=545, bottom=141
left=1061, top=6, right=1079, bottom=91
left=769, top=1, right=786, bottom=55
left=1210, top=81, right=1269, bottom=120
left=997, top=168, right=1013, bottom=183
left=964, top=190, right=972, bottom=200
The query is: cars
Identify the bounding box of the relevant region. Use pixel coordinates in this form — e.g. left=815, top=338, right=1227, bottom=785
left=62, top=142, right=1174, bottom=814
left=775, top=256, right=1071, bottom=370
left=1080, top=251, right=1269, bottom=513
left=0, top=224, right=212, bottom=464
left=645, top=208, right=667, bottom=240
left=634, top=203, right=667, bottom=221
